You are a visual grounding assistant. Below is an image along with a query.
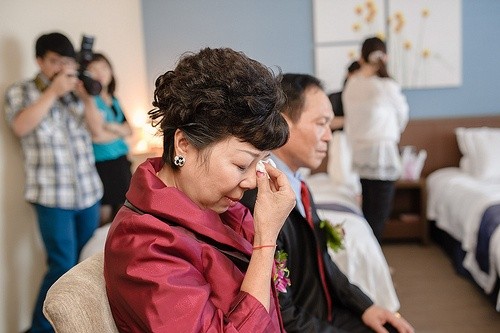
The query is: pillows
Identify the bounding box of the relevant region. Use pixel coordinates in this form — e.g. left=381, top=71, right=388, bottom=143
left=456, top=126, right=500, bottom=182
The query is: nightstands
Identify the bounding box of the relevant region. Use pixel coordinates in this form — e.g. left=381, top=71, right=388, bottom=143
left=383, top=176, right=427, bottom=243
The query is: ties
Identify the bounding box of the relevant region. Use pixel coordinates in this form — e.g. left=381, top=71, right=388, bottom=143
left=300, top=181, right=333, bottom=320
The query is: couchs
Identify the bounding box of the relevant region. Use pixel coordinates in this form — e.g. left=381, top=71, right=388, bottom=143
left=42, top=223, right=120, bottom=333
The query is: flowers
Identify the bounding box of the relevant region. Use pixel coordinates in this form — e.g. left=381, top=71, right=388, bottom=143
left=320, top=219, right=347, bottom=253
left=272, top=251, right=292, bottom=296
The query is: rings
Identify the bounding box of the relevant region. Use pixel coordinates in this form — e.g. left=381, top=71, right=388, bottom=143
left=395, top=312, right=402, bottom=318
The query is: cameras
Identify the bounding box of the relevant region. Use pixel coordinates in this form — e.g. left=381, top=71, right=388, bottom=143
left=77, top=36, right=102, bottom=95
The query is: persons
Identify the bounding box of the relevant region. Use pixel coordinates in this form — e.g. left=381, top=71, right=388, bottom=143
left=5, top=32, right=106, bottom=333
left=327, top=61, right=361, bottom=135
left=342, top=37, right=410, bottom=247
left=103, top=47, right=298, bottom=333
left=82, top=53, right=134, bottom=228
left=240, top=73, right=415, bottom=333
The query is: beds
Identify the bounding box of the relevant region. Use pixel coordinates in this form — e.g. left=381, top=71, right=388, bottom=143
left=305, top=147, right=401, bottom=314
left=401, top=117, right=500, bottom=312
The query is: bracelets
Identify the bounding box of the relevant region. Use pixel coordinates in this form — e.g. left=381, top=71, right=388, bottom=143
left=252, top=244, right=277, bottom=251
